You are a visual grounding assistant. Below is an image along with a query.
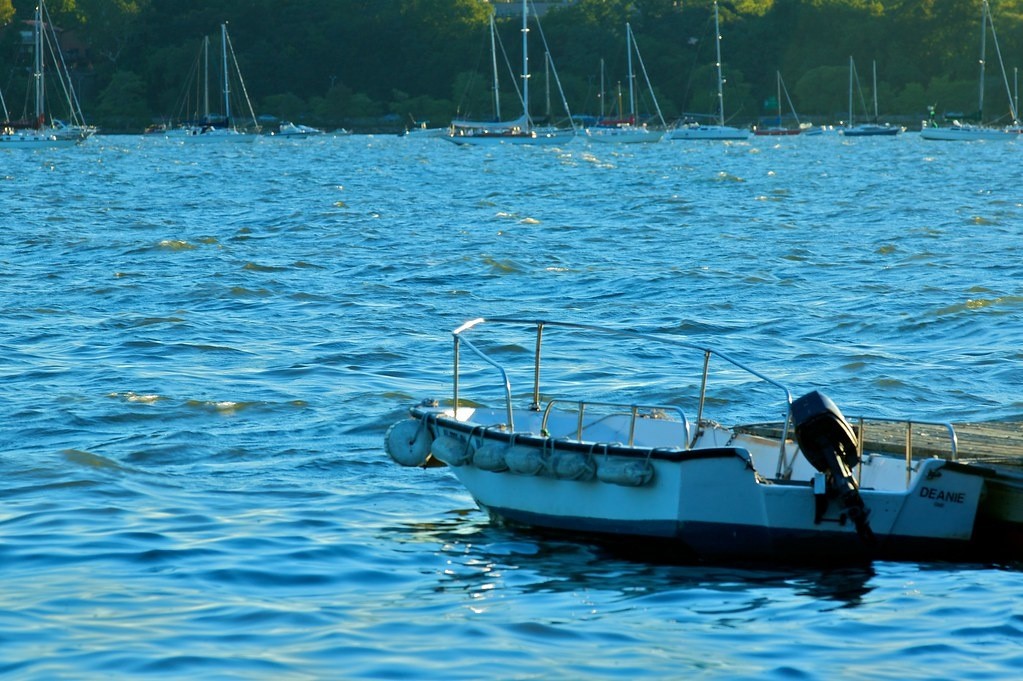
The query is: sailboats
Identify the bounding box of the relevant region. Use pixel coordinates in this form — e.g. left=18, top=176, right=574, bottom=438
left=143, top=1, right=1022, bottom=147
left=0, top=0, right=100, bottom=149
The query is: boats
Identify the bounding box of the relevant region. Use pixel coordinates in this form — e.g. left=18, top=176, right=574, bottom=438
left=385, top=316, right=999, bottom=554
